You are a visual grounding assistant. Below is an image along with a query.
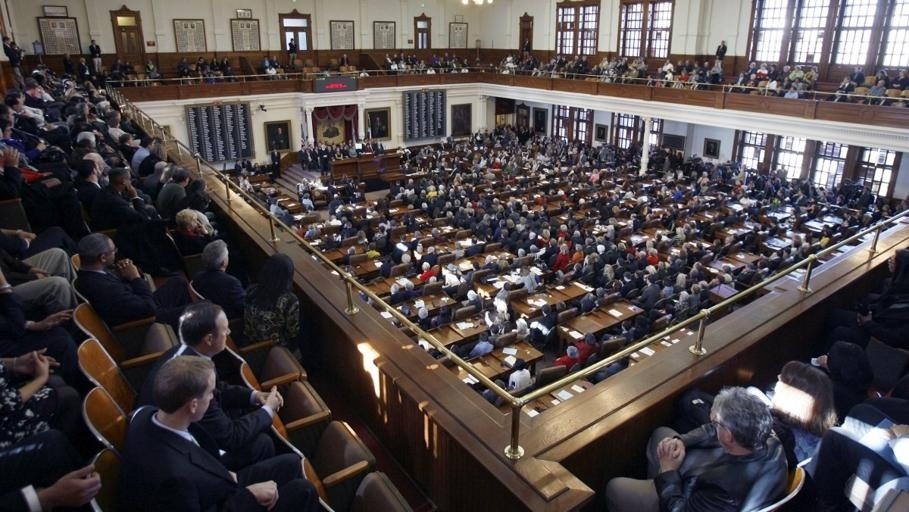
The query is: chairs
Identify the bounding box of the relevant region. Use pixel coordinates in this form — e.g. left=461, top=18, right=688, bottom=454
left=399, top=322, right=419, bottom=336
left=390, top=199, right=403, bottom=207
left=72, top=303, right=172, bottom=371
left=302, top=67, right=312, bottom=74
left=312, top=189, right=327, bottom=207
left=505, top=381, right=533, bottom=398
left=753, top=465, right=806, bottom=512
left=134, top=64, right=147, bottom=83
left=587, top=352, right=599, bottom=364
left=423, top=281, right=445, bottom=296
left=85, top=448, right=120, bottom=512
left=328, top=57, right=337, bottom=65
left=349, top=254, right=368, bottom=263
left=453, top=305, right=476, bottom=320
left=410, top=326, right=412, bottom=327
left=390, top=263, right=409, bottom=278
left=293, top=58, right=303, bottom=67
left=348, top=65, right=356, bottom=71
left=81, top=386, right=130, bottom=458
left=313, top=67, right=320, bottom=74
left=557, top=307, right=579, bottom=324
left=220, top=340, right=308, bottom=392
left=569, top=363, right=582, bottom=372
left=470, top=268, right=492, bottom=283
left=654, top=314, right=673, bottom=326
left=71, top=277, right=165, bottom=344
left=437, top=254, right=457, bottom=267
left=433, top=218, right=450, bottom=228
left=420, top=314, right=490, bottom=348
left=372, top=296, right=392, bottom=310
left=419, top=237, right=435, bottom=247
left=325, top=225, right=342, bottom=234
left=239, top=362, right=333, bottom=433
left=301, top=458, right=414, bottom=511
left=538, top=365, right=568, bottom=383
left=390, top=225, right=407, bottom=237
left=352, top=207, right=367, bottom=218
left=187, top=279, right=208, bottom=306
left=341, top=235, right=358, bottom=247
left=258, top=421, right=383, bottom=488
left=507, top=287, right=529, bottom=302
left=603, top=291, right=621, bottom=303
left=370, top=215, right=385, bottom=226
left=407, top=209, right=421, bottom=216
left=454, top=230, right=472, bottom=240
left=339, top=66, right=347, bottom=72
left=216, top=326, right=275, bottom=356
left=69, top=254, right=81, bottom=274
left=305, top=58, right=314, bottom=67
left=302, top=215, right=316, bottom=224
left=485, top=242, right=504, bottom=253
left=495, top=332, right=518, bottom=348
left=517, top=256, right=535, bottom=267
left=76, top=338, right=140, bottom=423
left=601, top=336, right=628, bottom=353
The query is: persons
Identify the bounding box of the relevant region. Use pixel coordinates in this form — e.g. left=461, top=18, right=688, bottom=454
left=772, top=361, right=837, bottom=438
left=851, top=249, right=909, bottom=317
left=0, top=457, right=101, bottom=511
left=304, top=127, right=906, bottom=249
left=134, top=303, right=284, bottom=467
left=0, top=226, right=73, bottom=258
left=817, top=341, right=864, bottom=381
left=0, top=265, right=74, bottom=355
left=732, top=61, right=818, bottom=98
left=176, top=209, right=226, bottom=254
left=78, top=159, right=102, bottom=210
left=346, top=250, right=614, bottom=400
left=0, top=234, right=72, bottom=316
left=715, top=40, right=727, bottom=68
left=522, top=37, right=530, bottom=51
left=126, top=356, right=319, bottom=512
left=177, top=55, right=231, bottom=83
left=156, top=169, right=190, bottom=218
left=0, top=348, right=78, bottom=439
left=288, top=39, right=297, bottom=65
left=806, top=403, right=909, bottom=488
left=272, top=127, right=288, bottom=150
left=829, top=65, right=909, bottom=105
left=0, top=147, right=82, bottom=237
left=0, top=36, right=167, bottom=175
left=160, top=148, right=185, bottom=184
left=187, top=191, right=210, bottom=210
left=222, top=149, right=303, bottom=238
left=79, top=231, right=195, bottom=332
left=262, top=55, right=284, bottom=80
left=341, top=54, right=348, bottom=66
left=499, top=49, right=719, bottom=90
left=828, top=310, right=909, bottom=349
left=193, top=241, right=243, bottom=316
left=606, top=389, right=772, bottom=512
left=89, top=168, right=145, bottom=230
left=382, top=48, right=482, bottom=74
left=614, top=250, right=806, bottom=341
left=241, top=255, right=301, bottom=350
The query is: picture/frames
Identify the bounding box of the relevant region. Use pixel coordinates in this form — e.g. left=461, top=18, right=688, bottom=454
left=172, top=18, right=208, bottom=52
left=373, top=19, right=396, bottom=49
left=329, top=19, right=355, bottom=49
left=264, top=119, right=295, bottom=154
left=448, top=21, right=469, bottom=48
left=363, top=106, right=393, bottom=142
left=36, top=16, right=83, bottom=55
left=230, top=18, right=262, bottom=52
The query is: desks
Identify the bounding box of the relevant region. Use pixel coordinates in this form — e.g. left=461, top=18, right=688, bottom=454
left=714, top=219, right=754, bottom=244
left=342, top=259, right=384, bottom=276
left=762, top=229, right=802, bottom=257
left=556, top=299, right=644, bottom=348
left=696, top=209, right=724, bottom=221
left=763, top=201, right=794, bottom=222
left=587, top=217, right=631, bottom=236
left=498, top=378, right=594, bottom=418
left=328, top=151, right=400, bottom=178
left=550, top=208, right=588, bottom=229
left=621, top=226, right=666, bottom=247
left=274, top=194, right=305, bottom=214
left=509, top=284, right=588, bottom=318
left=448, top=342, right=543, bottom=385
left=357, top=276, right=428, bottom=295
left=399, top=228, right=447, bottom=247
left=471, top=268, right=547, bottom=298
left=390, top=293, right=457, bottom=319
left=382, top=206, right=416, bottom=220
left=446, top=250, right=516, bottom=273
left=719, top=201, right=746, bottom=217
left=668, top=236, right=712, bottom=264
left=802, top=214, right=844, bottom=245
left=704, top=250, right=762, bottom=278
left=543, top=200, right=575, bottom=217
left=429, top=237, right=478, bottom=255
left=248, top=174, right=281, bottom=195
left=321, top=244, right=366, bottom=262
left=676, top=213, right=709, bottom=230
left=626, top=324, right=694, bottom=369
